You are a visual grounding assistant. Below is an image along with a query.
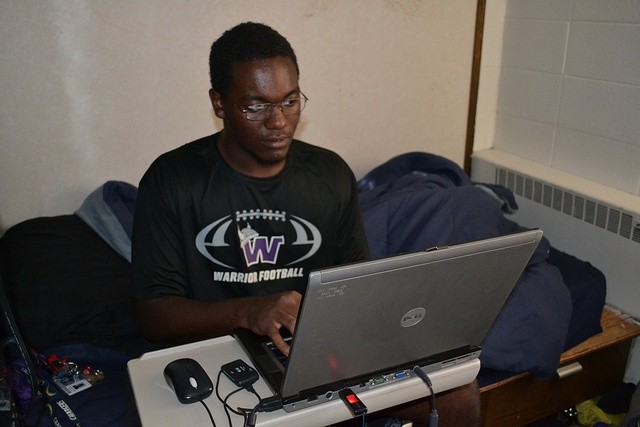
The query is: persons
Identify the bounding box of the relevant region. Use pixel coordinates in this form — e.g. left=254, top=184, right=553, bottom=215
left=129, top=22, right=371, bottom=359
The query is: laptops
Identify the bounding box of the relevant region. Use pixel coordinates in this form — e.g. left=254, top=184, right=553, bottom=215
left=233, top=228, right=543, bottom=414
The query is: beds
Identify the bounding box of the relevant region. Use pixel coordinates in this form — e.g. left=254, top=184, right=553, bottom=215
left=0, top=150, right=639, bottom=425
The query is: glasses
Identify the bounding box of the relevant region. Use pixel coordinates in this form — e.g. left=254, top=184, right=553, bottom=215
left=218, top=90, right=309, bottom=121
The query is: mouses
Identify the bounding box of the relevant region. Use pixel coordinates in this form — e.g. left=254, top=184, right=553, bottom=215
left=163, top=357, right=213, bottom=404
